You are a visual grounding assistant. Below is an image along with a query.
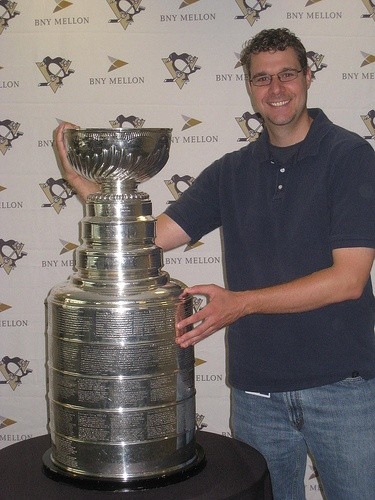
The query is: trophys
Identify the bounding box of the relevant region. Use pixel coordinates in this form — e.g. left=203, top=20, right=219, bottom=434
left=45, top=127, right=206, bottom=491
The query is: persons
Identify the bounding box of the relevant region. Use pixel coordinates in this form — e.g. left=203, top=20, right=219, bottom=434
left=50, top=26, right=374, bottom=500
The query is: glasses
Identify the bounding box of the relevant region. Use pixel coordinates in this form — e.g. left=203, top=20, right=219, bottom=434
left=247, top=66, right=306, bottom=86
